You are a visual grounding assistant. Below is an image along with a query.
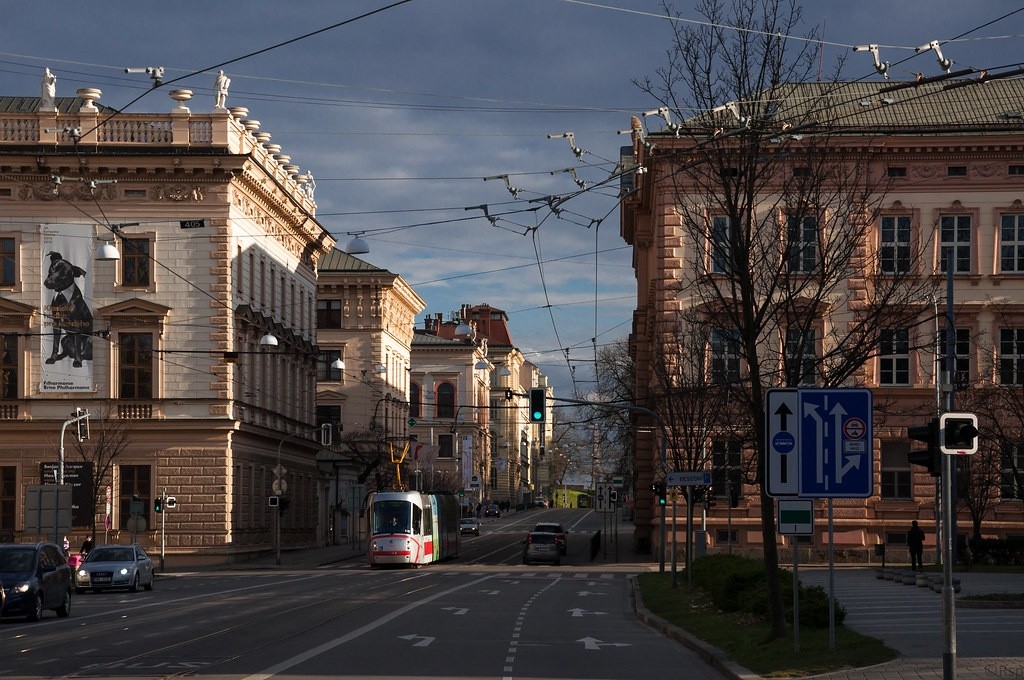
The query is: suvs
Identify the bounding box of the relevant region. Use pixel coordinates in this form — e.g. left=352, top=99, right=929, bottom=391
left=0, top=541, right=72, bottom=621
left=75, top=542, right=155, bottom=592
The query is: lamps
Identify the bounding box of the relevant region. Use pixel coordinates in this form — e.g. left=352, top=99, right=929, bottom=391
left=503, top=441, right=582, bottom=467
left=500, top=366, right=511, bottom=376
left=376, top=364, right=388, bottom=373
left=475, top=358, right=489, bottom=369
left=346, top=231, right=369, bottom=254
left=94, top=238, right=120, bottom=261
left=259, top=333, right=279, bottom=346
left=331, top=358, right=345, bottom=369
left=455, top=320, right=472, bottom=336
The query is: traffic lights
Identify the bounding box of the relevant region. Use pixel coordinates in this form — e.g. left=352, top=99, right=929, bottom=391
left=154, top=498, right=163, bottom=512
left=268, top=497, right=279, bottom=506
left=907, top=416, right=941, bottom=476
left=705, top=490, right=718, bottom=509
left=660, top=484, right=666, bottom=505
left=611, top=491, right=617, bottom=501
left=71, top=407, right=84, bottom=442
left=167, top=497, right=176, bottom=507
left=941, top=413, right=979, bottom=454
left=529, top=388, right=546, bottom=423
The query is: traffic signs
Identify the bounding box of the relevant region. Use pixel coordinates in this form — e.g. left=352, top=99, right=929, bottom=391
left=663, top=471, right=712, bottom=486
left=798, top=388, right=874, bottom=498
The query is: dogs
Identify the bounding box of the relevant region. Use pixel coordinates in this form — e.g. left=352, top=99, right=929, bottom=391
left=44, top=250, right=92, bottom=368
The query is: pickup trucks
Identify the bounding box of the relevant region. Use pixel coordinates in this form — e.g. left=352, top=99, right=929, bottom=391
left=534, top=497, right=544, bottom=507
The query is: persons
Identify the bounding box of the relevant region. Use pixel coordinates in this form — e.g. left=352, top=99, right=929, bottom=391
left=500, top=499, right=510, bottom=512
left=476, top=503, right=481, bottom=519
left=64, top=535, right=70, bottom=549
left=469, top=503, right=473, bottom=518
left=387, top=517, right=400, bottom=528
left=907, top=521, right=925, bottom=573
left=80, top=534, right=92, bottom=557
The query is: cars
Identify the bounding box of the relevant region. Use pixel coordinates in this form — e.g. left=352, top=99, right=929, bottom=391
left=460, top=518, right=480, bottom=536
left=523, top=522, right=569, bottom=565
left=485, top=504, right=500, bottom=518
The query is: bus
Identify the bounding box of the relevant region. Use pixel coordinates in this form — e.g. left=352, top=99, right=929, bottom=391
left=364, top=440, right=461, bottom=568
